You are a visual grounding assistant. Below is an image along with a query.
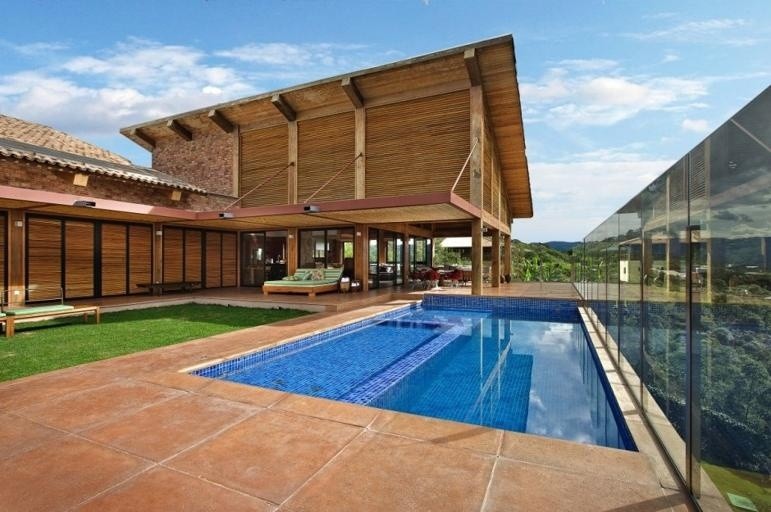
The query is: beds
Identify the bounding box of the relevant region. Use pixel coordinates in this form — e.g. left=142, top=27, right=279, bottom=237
left=262, top=268, right=342, bottom=296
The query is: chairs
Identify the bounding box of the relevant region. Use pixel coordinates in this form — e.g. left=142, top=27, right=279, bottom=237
left=412, top=267, right=474, bottom=291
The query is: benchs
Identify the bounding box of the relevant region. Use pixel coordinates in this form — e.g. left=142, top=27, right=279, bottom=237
left=1, top=305, right=100, bottom=338
left=136, top=281, right=201, bottom=296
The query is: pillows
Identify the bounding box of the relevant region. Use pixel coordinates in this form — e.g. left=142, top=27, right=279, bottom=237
left=282, top=275, right=298, bottom=281
left=311, top=270, right=324, bottom=280
left=303, top=272, right=310, bottom=280
